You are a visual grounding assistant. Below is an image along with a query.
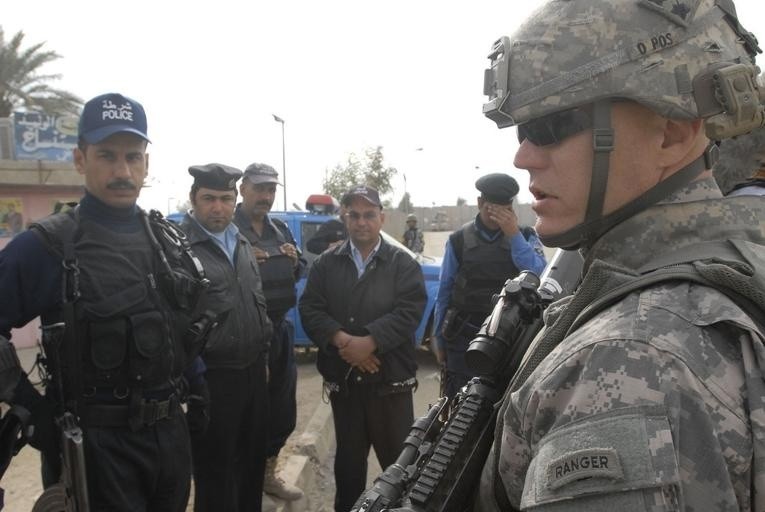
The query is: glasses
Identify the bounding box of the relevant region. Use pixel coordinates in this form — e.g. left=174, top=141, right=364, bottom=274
left=518, top=108, right=588, bottom=146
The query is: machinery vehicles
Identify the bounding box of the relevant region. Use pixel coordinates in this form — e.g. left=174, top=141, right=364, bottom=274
left=431, top=211, right=453, bottom=233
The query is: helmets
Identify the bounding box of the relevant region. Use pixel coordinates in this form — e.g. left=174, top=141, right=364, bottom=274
left=480, top=1, right=764, bottom=142
left=406, top=214, right=417, bottom=222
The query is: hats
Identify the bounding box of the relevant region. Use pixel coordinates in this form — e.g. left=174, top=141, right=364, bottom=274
left=79, top=91, right=152, bottom=144
left=341, top=185, right=383, bottom=207
left=475, top=172, right=520, bottom=205
left=189, top=163, right=243, bottom=192
left=243, top=163, right=279, bottom=185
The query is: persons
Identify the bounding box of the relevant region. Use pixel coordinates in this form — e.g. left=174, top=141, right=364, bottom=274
left=403, top=213, right=425, bottom=256
left=176, top=163, right=273, bottom=512
left=298, top=185, right=428, bottom=512
left=3, top=204, right=23, bottom=235
left=432, top=173, right=546, bottom=397
left=459, top=1, right=765, bottom=511
left=231, top=163, right=308, bottom=502
left=1, top=93, right=213, bottom=508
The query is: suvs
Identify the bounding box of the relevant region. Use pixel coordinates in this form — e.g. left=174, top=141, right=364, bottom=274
left=160, top=192, right=444, bottom=370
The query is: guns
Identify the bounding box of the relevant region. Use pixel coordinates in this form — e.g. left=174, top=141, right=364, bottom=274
left=349, top=248, right=584, bottom=511
left=188, top=310, right=218, bottom=357
left=32, top=323, right=90, bottom=511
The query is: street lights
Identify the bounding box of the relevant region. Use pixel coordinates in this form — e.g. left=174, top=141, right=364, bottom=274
left=270, top=112, right=292, bottom=210
left=167, top=196, right=176, bottom=216
left=401, top=146, right=425, bottom=216
